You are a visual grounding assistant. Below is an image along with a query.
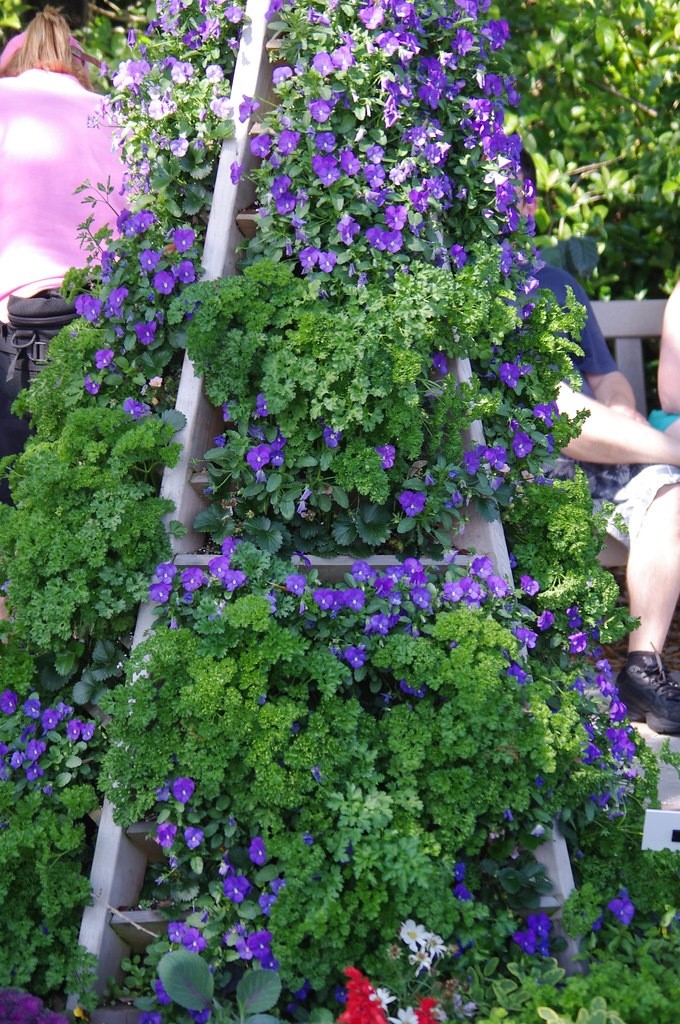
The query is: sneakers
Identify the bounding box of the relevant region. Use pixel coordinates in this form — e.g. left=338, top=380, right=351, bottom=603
left=614, top=641, right=680, bottom=733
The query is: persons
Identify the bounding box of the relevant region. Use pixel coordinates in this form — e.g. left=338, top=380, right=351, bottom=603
left=649, top=279, right=680, bottom=438
left=511, top=149, right=680, bottom=735
left=0, top=4, right=137, bottom=506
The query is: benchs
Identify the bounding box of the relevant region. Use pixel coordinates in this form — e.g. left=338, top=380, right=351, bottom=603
left=583, top=303, right=670, bottom=567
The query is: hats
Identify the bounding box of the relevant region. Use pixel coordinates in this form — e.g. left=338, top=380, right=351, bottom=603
left=0, top=30, right=101, bottom=76
